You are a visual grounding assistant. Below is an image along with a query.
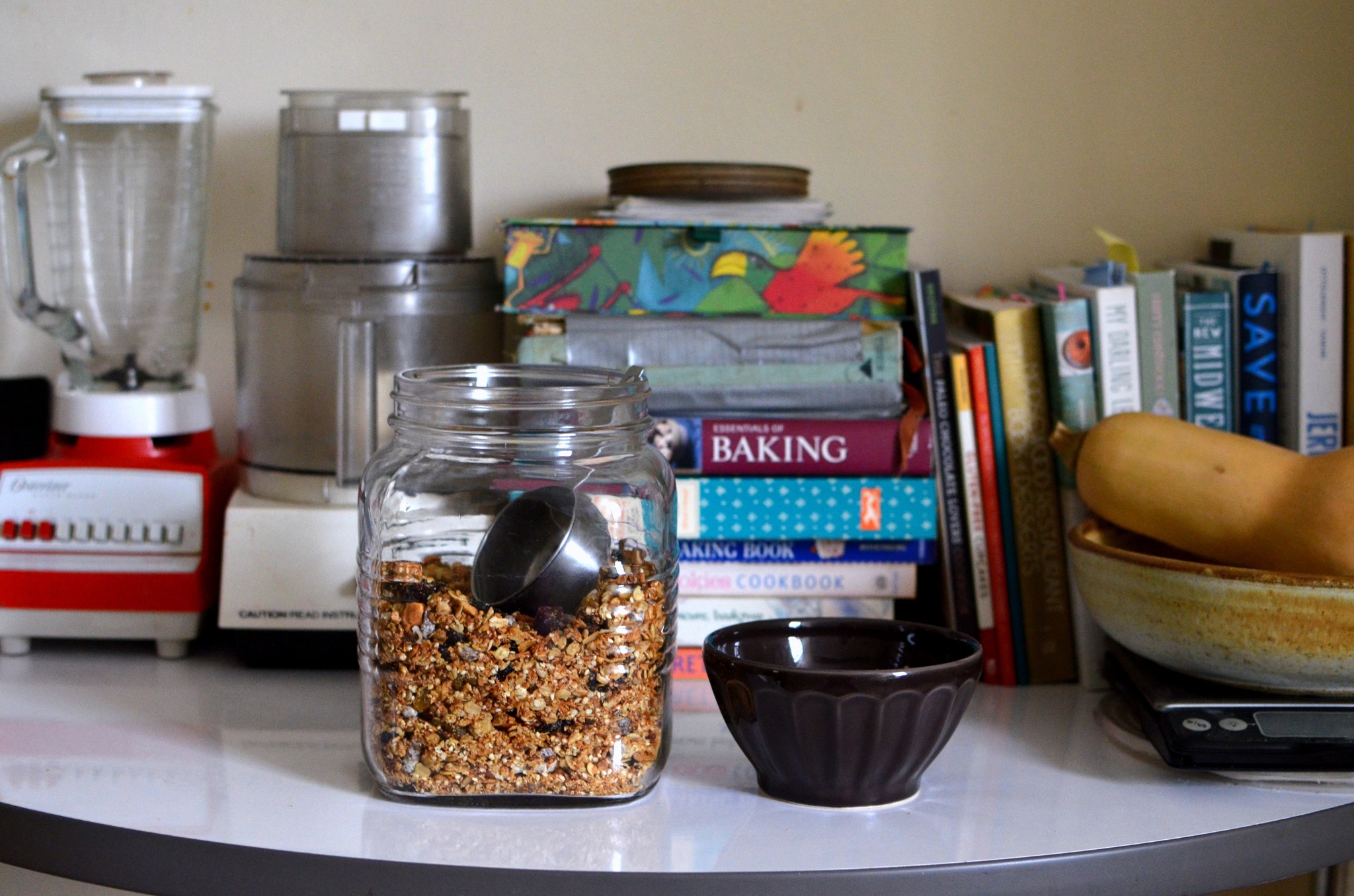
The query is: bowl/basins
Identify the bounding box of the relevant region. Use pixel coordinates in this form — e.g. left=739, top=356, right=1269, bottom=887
left=1069, top=512, right=1354, bottom=692
left=702, top=617, right=985, bottom=809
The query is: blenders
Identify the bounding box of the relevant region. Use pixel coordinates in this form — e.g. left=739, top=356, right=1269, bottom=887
left=216, top=89, right=508, bottom=667
left=0, top=71, right=235, bottom=660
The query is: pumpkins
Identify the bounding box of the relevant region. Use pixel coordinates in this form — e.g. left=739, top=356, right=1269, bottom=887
left=1051, top=411, right=1354, bottom=582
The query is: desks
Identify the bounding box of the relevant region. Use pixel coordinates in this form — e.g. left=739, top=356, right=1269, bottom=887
left=0, top=651, right=1354, bottom=896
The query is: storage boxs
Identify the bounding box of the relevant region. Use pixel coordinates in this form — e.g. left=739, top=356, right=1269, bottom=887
left=494, top=217, right=913, bottom=322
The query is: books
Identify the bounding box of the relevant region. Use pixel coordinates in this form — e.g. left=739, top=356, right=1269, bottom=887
left=496, top=197, right=1354, bottom=690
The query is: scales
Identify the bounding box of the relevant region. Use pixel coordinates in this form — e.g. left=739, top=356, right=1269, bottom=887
left=1097, top=642, right=1352, bottom=770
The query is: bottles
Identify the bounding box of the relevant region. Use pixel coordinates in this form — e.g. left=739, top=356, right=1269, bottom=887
left=357, top=362, right=679, bottom=799
